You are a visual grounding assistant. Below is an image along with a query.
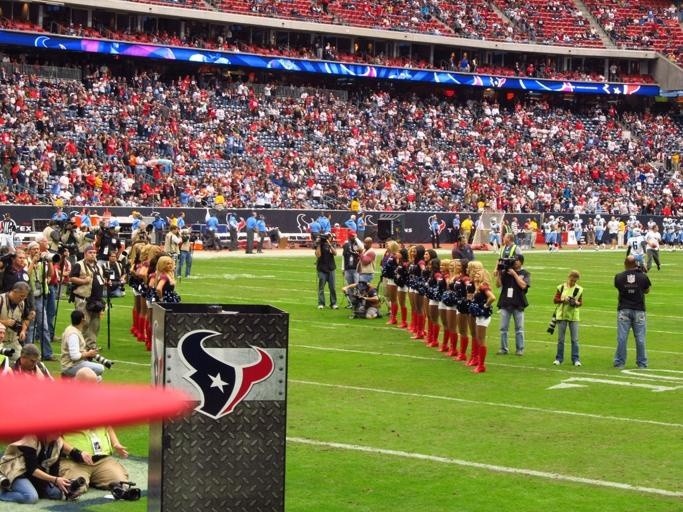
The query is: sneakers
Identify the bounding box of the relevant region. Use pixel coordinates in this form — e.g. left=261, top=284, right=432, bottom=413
left=329, top=305, right=339, bottom=310
left=47, top=357, right=57, bottom=361
left=50, top=335, right=61, bottom=343
left=515, top=349, right=523, bottom=355
left=553, top=361, right=560, bottom=365
left=344, top=304, right=352, bottom=309
left=376, top=312, right=382, bottom=318
left=497, top=350, right=509, bottom=355
left=318, top=305, right=324, bottom=309
left=616, top=366, right=624, bottom=369
left=636, top=364, right=648, bottom=368
left=574, top=361, right=581, bottom=366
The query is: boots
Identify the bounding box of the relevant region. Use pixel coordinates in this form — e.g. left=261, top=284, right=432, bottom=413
left=397, top=307, right=407, bottom=328
left=137, top=317, right=147, bottom=341
left=145, top=320, right=150, bottom=346
left=453, top=336, right=468, bottom=361
left=438, top=330, right=449, bottom=352
left=427, top=324, right=440, bottom=347
left=447, top=332, right=458, bottom=357
left=465, top=337, right=479, bottom=366
left=146, top=327, right=151, bottom=351
left=420, top=320, right=433, bottom=343
left=471, top=346, right=487, bottom=373
left=410, top=313, right=427, bottom=339
left=130, top=308, right=137, bottom=334
left=407, top=312, right=417, bottom=333
left=387, top=303, right=398, bottom=324
left=134, top=311, right=139, bottom=337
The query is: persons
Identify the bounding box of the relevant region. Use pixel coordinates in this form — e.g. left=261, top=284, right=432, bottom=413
left=57, top=247, right=71, bottom=284
left=0, top=212, right=17, bottom=251
left=645, top=224, right=662, bottom=271
left=470, top=270, right=496, bottom=373
left=177, top=227, right=192, bottom=279
left=67, top=246, right=114, bottom=349
left=11, top=344, right=55, bottom=380
left=496, top=254, right=531, bottom=356
left=0, top=432, right=71, bottom=504
left=614, top=255, right=651, bottom=370
left=164, top=225, right=182, bottom=280
left=129, top=243, right=181, bottom=351
left=43, top=208, right=154, bottom=260
left=381, top=238, right=398, bottom=325
left=342, top=281, right=383, bottom=319
left=101, top=251, right=125, bottom=297
left=0, top=355, right=13, bottom=375
left=342, top=231, right=364, bottom=309
left=626, top=216, right=642, bottom=239
left=35, top=232, right=62, bottom=342
left=465, top=262, right=482, bottom=367
left=0, top=0, right=683, bottom=82
left=356, top=237, right=376, bottom=284
left=0, top=322, right=9, bottom=375
left=4, top=248, right=36, bottom=348
left=452, top=234, right=474, bottom=262
left=152, top=212, right=186, bottom=245
left=663, top=217, right=683, bottom=250
left=300, top=212, right=366, bottom=249
left=60, top=310, right=105, bottom=383
left=540, top=215, right=628, bottom=251
left=408, top=245, right=482, bottom=366
left=315, top=232, right=339, bottom=310
left=206, top=212, right=280, bottom=254
left=454, top=259, right=470, bottom=361
left=395, top=249, right=408, bottom=328
left=58, top=367, right=129, bottom=496
left=489, top=214, right=538, bottom=276
left=553, top=270, right=584, bottom=366
left=452, top=214, right=482, bottom=244
left=647, top=218, right=656, bottom=231
left=0, top=281, right=31, bottom=361
left=24, top=242, right=59, bottom=362
left=0, top=63, right=683, bottom=216
left=626, top=228, right=647, bottom=273
left=430, top=214, right=442, bottom=248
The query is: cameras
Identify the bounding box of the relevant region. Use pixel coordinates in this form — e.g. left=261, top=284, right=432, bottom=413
left=9, top=321, right=23, bottom=336
left=41, top=252, right=60, bottom=263
left=309, top=231, right=329, bottom=243
left=66, top=475, right=86, bottom=500
left=104, top=269, right=114, bottom=278
left=108, top=480, right=140, bottom=503
left=93, top=352, right=114, bottom=369
left=1, top=245, right=17, bottom=272
left=0, top=347, right=16, bottom=357
left=58, top=241, right=77, bottom=253
left=498, top=256, right=515, bottom=274
left=547, top=312, right=559, bottom=335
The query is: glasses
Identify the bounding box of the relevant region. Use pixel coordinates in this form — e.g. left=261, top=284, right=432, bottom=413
left=14, top=294, right=26, bottom=301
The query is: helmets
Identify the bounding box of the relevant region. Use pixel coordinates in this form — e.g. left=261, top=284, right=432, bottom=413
left=668, top=218, right=672, bottom=224
left=663, top=218, right=668, bottom=223
left=633, top=228, right=641, bottom=237
left=630, top=216, right=634, bottom=221
left=549, top=215, right=554, bottom=220
left=596, top=215, right=600, bottom=219
left=681, top=220, right=683, bottom=225
left=491, top=218, right=496, bottom=222
left=575, top=214, right=579, bottom=219
left=633, top=216, right=636, bottom=221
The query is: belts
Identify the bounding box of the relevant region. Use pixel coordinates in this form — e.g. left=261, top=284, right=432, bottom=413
left=74, top=293, right=87, bottom=301
left=34, top=293, right=43, bottom=299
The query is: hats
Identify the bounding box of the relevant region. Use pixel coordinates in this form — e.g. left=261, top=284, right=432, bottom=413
left=5, top=213, right=10, bottom=217
left=348, top=231, right=355, bottom=238
left=36, top=234, right=44, bottom=243
left=75, top=367, right=102, bottom=383
left=358, top=281, right=368, bottom=290
left=324, top=232, right=331, bottom=237
left=515, top=254, right=524, bottom=265
left=108, top=224, right=115, bottom=228
left=28, top=241, right=36, bottom=250
left=559, top=217, right=564, bottom=219
left=84, top=246, right=95, bottom=253
